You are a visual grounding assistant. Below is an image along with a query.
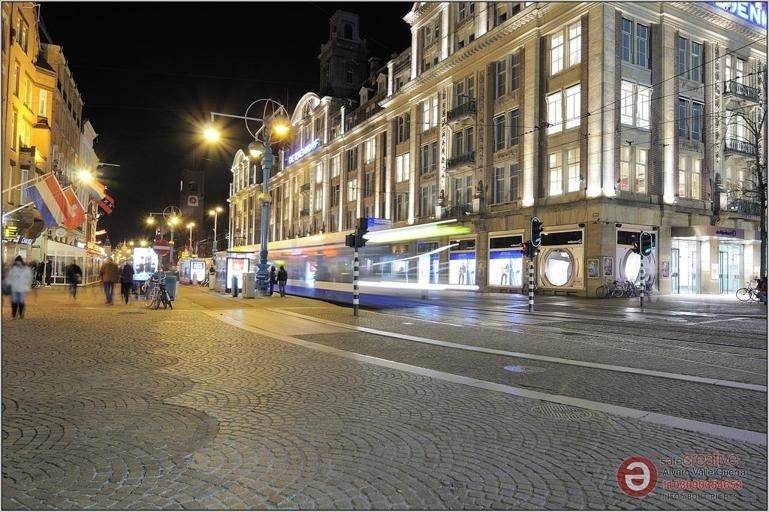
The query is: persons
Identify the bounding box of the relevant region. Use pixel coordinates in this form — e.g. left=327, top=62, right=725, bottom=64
left=276, top=265, right=287, bottom=298
left=209, top=264, right=215, bottom=273
left=98, top=257, right=135, bottom=304
left=62, top=258, right=83, bottom=304
left=269, top=266, right=276, bottom=297
left=499, top=261, right=508, bottom=285
left=457, top=261, right=466, bottom=284
left=1, top=255, right=52, bottom=321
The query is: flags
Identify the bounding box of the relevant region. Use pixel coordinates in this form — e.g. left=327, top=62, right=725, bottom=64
left=84, top=177, right=106, bottom=202
left=62, top=187, right=87, bottom=230
left=91, top=194, right=114, bottom=214
left=24, top=175, right=72, bottom=230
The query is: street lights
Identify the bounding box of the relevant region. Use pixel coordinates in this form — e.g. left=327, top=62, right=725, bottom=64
left=203, top=99, right=291, bottom=297
left=186, top=221, right=196, bottom=254
left=146, top=206, right=183, bottom=265
left=113, top=239, right=155, bottom=267
left=208, top=206, right=223, bottom=253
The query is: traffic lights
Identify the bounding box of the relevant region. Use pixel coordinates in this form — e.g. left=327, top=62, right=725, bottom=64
left=532, top=247, right=540, bottom=258
left=531, top=216, right=543, bottom=247
left=640, top=231, right=652, bottom=257
left=630, top=232, right=640, bottom=254
left=360, top=237, right=370, bottom=248
left=520, top=240, right=530, bottom=256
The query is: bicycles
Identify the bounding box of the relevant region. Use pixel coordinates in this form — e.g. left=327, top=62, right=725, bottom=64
left=31, top=277, right=41, bottom=288
left=736, top=280, right=767, bottom=302
left=144, top=275, right=167, bottom=310
left=595, top=276, right=647, bottom=299
left=749, top=285, right=767, bottom=302
left=201, top=274, right=210, bottom=287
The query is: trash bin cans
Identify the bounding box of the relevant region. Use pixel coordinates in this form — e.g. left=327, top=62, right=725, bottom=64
left=165, top=276, right=177, bottom=301
left=208, top=272, right=216, bottom=290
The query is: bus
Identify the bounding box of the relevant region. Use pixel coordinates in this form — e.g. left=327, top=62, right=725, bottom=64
left=224, top=229, right=430, bottom=309
